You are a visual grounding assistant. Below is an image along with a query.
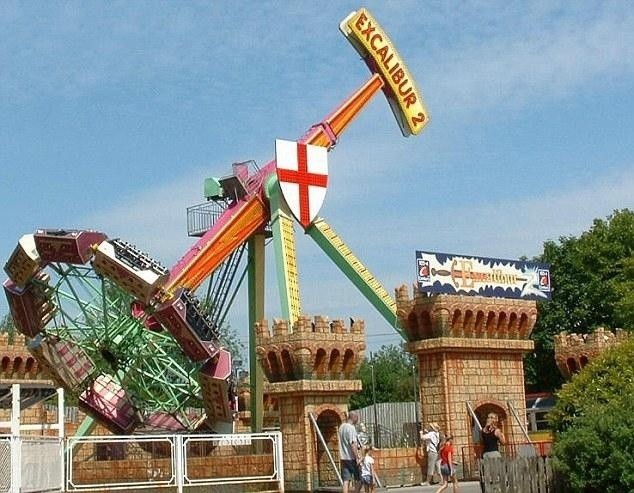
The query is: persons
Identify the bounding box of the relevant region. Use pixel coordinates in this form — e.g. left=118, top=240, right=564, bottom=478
left=433, top=435, right=457, bottom=493
left=479, top=411, right=506, bottom=493
left=417, top=422, right=445, bottom=486
left=336, top=410, right=364, bottom=492
left=354, top=444, right=376, bottom=492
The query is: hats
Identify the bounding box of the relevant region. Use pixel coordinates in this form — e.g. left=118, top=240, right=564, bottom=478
left=429, top=422, right=441, bottom=432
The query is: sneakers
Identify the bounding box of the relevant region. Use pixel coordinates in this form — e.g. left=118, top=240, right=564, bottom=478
left=420, top=480, right=445, bottom=486
